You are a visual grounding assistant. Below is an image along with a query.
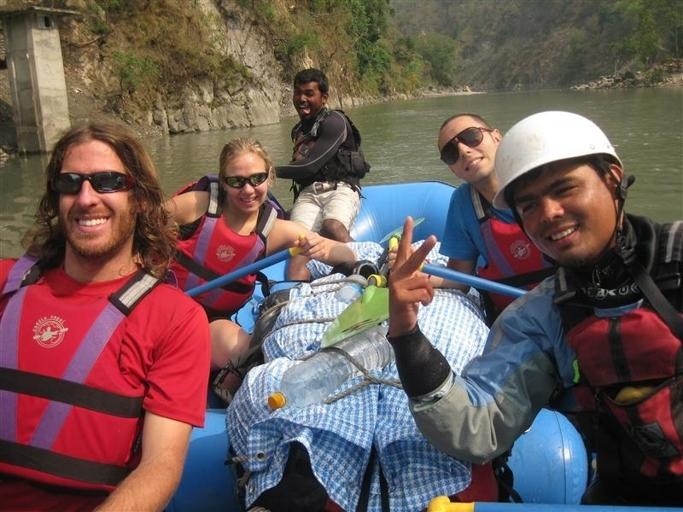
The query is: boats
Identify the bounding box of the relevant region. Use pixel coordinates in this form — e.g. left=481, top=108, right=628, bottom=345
left=164, top=174, right=588, bottom=512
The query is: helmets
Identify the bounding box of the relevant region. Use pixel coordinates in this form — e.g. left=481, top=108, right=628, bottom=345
left=490, top=109, right=626, bottom=212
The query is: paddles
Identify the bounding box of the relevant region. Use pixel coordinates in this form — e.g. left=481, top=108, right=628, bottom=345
left=319, top=218, right=424, bottom=350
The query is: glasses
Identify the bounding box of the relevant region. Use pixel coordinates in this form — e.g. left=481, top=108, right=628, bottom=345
left=439, top=125, right=493, bottom=166
left=51, top=170, right=137, bottom=196
left=221, top=171, right=270, bottom=189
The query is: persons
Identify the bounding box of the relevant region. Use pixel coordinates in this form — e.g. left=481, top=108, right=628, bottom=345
left=385, top=110, right=683, bottom=508
left=1, top=115, right=212, bottom=511
left=142, top=136, right=355, bottom=374
left=273, top=66, right=373, bottom=281
left=386, top=111, right=558, bottom=329
